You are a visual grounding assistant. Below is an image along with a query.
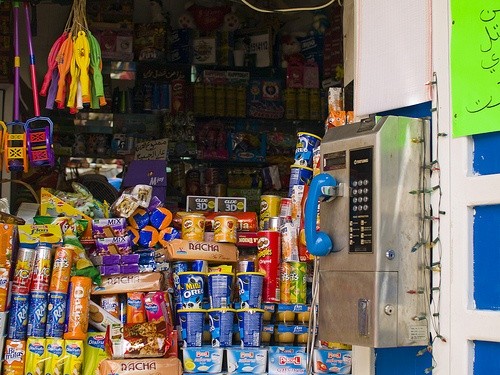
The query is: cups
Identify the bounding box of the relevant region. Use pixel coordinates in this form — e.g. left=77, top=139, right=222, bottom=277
left=208, top=271, right=235, bottom=309
left=236, top=271, right=266, bottom=309
left=288, top=164, right=314, bottom=199
left=293, top=132, right=322, bottom=168
left=177, top=270, right=206, bottom=309
left=176, top=309, right=207, bottom=349
left=236, top=309, right=265, bottom=348
left=207, top=309, right=236, bottom=348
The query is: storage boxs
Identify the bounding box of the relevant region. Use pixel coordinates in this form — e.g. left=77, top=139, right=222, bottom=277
left=0, top=222, right=17, bottom=375
left=180, top=344, right=353, bottom=375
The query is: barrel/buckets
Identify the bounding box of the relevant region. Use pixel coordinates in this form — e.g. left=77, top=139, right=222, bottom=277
left=181, top=215, right=206, bottom=241
left=259, top=194, right=281, bottom=229
left=213, top=216, right=238, bottom=244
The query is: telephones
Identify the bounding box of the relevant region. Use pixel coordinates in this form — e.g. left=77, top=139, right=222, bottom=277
left=306, top=115, right=429, bottom=347
